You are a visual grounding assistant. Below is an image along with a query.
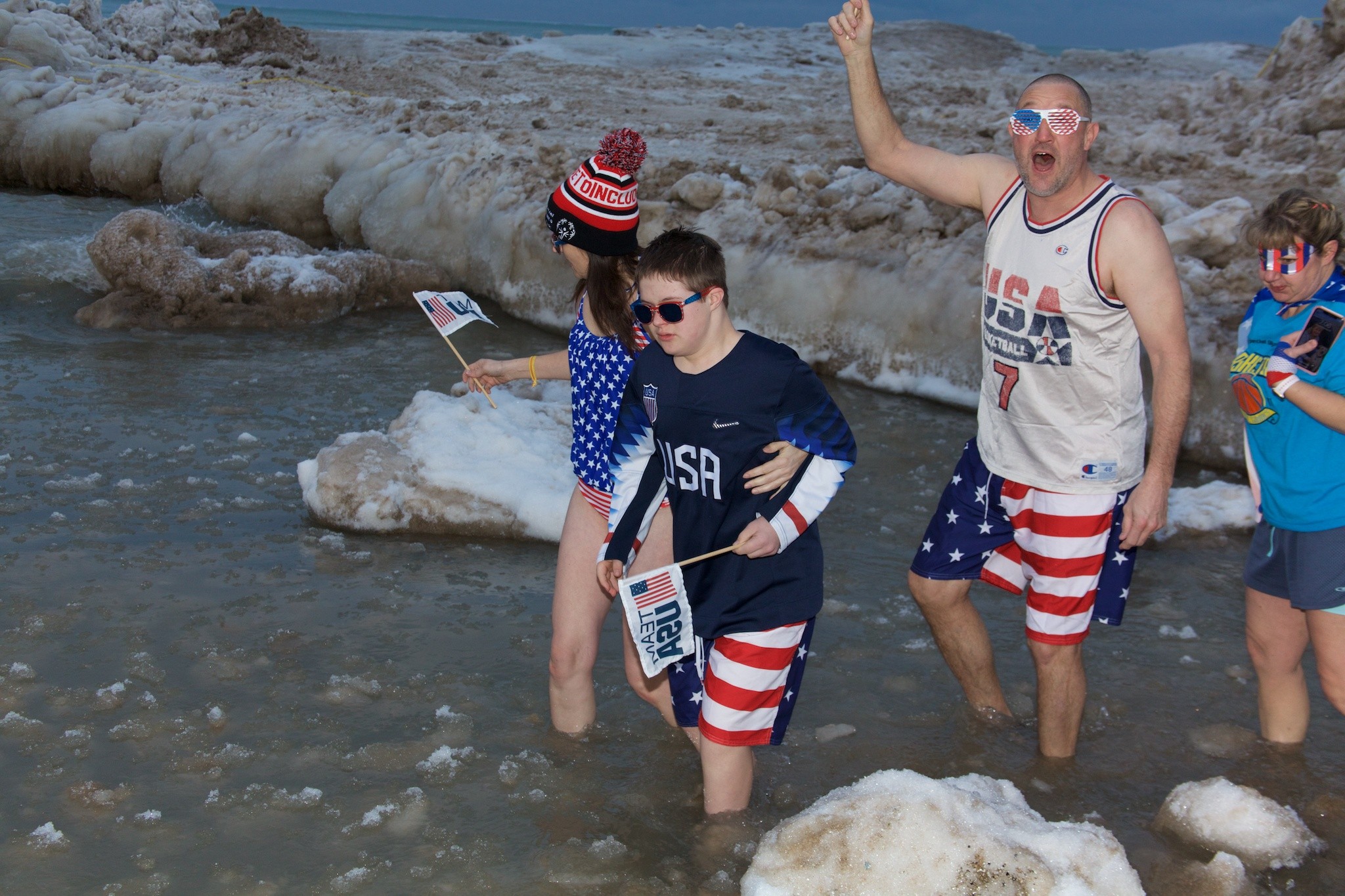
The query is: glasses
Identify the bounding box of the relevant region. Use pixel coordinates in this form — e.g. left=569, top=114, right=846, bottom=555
left=630, top=284, right=718, bottom=325
left=550, top=233, right=568, bottom=255
left=1009, top=109, right=1090, bottom=135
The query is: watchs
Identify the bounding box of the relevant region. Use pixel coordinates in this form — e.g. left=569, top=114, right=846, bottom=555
left=1272, top=375, right=1300, bottom=397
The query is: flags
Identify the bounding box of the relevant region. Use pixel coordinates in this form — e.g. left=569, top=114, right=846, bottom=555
left=414, top=291, right=499, bottom=337
left=618, top=565, right=695, bottom=678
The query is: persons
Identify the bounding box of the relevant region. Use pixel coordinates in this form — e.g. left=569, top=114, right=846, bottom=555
left=595, top=226, right=858, bottom=807
left=826, top=0, right=1191, bottom=754
left=1229, top=191, right=1345, bottom=740
left=463, top=130, right=807, bottom=732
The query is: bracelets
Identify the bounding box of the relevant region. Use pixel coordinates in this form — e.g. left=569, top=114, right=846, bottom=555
left=530, top=356, right=538, bottom=387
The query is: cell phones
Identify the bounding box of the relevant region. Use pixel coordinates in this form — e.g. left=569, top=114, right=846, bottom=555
left=1290, top=306, right=1345, bottom=376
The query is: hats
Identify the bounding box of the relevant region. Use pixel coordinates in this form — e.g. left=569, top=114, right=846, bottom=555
left=545, top=128, right=648, bottom=257
